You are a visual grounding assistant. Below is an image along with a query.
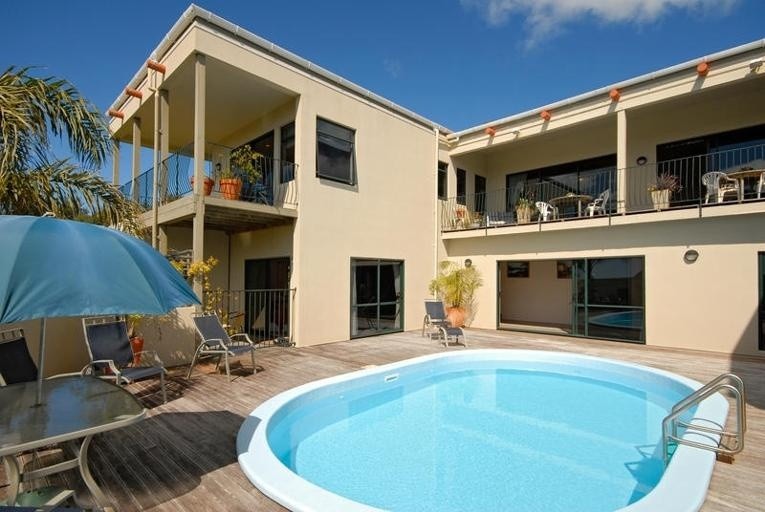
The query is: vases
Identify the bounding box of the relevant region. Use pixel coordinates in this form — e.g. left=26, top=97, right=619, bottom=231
left=190, top=176, right=215, bottom=195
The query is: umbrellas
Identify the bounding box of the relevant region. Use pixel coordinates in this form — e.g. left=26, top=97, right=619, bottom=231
left=0, top=213, right=199, bottom=407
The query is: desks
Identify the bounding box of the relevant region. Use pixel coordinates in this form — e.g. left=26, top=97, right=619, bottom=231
left=0, top=375, right=148, bottom=512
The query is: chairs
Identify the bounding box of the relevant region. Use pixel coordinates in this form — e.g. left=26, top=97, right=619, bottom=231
left=0, top=310, right=257, bottom=405
left=422, top=302, right=467, bottom=348
left=702, top=172, right=741, bottom=204
left=583, top=189, right=610, bottom=217
left=535, top=201, right=560, bottom=222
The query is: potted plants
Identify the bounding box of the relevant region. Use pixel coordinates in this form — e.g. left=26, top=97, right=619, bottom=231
left=647, top=174, right=684, bottom=210
left=516, top=197, right=530, bottom=224
left=102, top=254, right=224, bottom=377
left=220, top=169, right=244, bottom=201
left=428, top=261, right=480, bottom=329
